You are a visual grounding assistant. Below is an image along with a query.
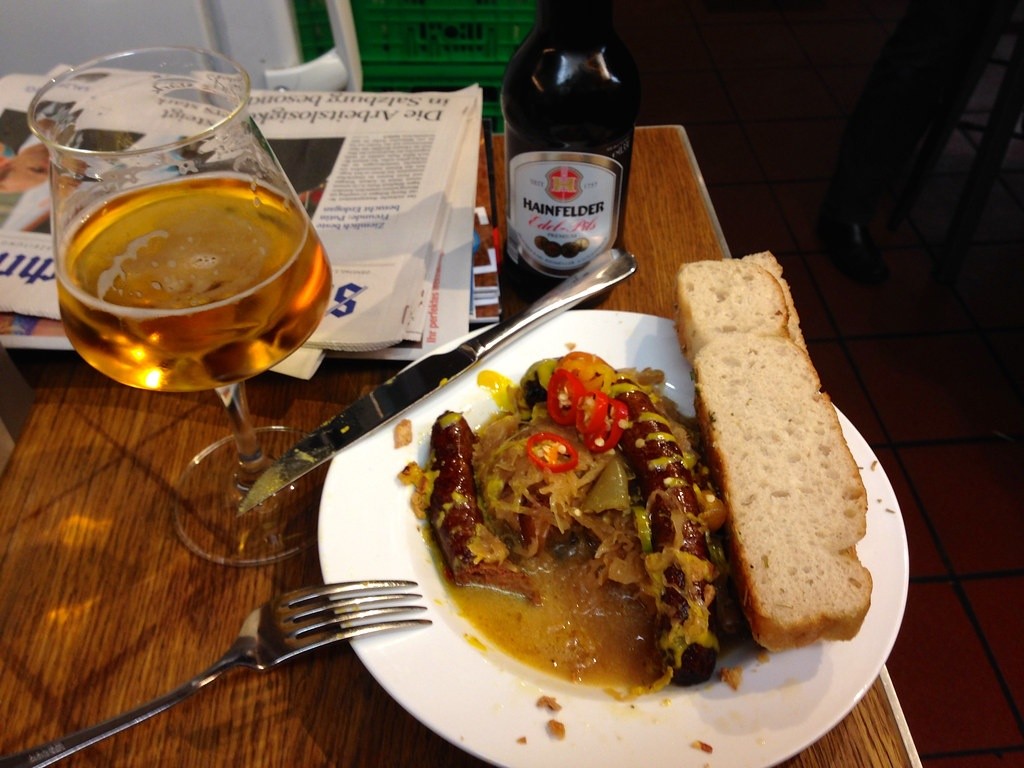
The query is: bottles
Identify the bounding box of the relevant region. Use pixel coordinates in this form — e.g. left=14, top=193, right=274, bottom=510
left=499, top=1, right=637, bottom=310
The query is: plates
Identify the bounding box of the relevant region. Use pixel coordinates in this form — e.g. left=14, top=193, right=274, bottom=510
left=317, top=309, right=910, bottom=768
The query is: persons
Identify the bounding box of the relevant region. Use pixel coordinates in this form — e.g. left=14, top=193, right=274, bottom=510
left=815, top=0, right=991, bottom=285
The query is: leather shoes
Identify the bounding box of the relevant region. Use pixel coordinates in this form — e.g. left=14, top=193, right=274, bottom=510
left=818, top=215, right=888, bottom=283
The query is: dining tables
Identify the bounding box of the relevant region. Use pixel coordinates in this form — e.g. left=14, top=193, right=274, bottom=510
left=0, top=127, right=925, bottom=766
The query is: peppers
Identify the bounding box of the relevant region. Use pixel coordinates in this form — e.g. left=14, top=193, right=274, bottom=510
left=527, top=367, right=651, bottom=551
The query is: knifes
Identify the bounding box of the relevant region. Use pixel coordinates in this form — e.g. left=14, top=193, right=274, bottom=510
left=238, top=248, right=637, bottom=524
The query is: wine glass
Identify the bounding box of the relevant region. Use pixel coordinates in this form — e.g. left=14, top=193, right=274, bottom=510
left=26, top=47, right=333, bottom=566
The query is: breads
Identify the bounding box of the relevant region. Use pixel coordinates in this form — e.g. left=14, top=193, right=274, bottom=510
left=671, top=250, right=872, bottom=651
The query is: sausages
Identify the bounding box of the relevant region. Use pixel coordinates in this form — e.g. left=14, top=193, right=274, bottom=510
left=426, top=413, right=541, bottom=605
left=612, top=373, right=716, bottom=686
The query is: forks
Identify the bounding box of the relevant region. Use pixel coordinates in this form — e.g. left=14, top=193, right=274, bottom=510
left=0, top=581, right=432, bottom=768
